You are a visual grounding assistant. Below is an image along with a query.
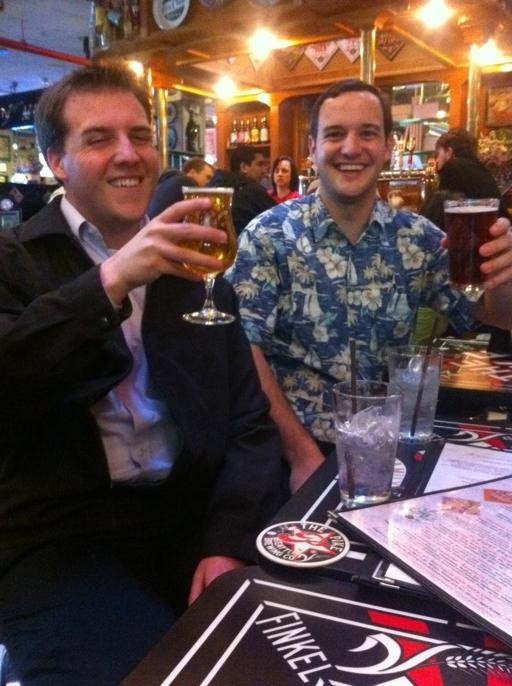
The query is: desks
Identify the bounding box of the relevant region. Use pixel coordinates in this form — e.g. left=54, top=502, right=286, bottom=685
left=237, top=412, right=511, bottom=597
left=406, top=328, right=512, bottom=393
left=115, top=564, right=512, bottom=686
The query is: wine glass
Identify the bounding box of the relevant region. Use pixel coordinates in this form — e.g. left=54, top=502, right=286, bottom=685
left=178, top=188, right=240, bottom=326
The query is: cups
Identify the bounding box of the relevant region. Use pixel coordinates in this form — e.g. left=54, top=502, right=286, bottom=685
left=390, top=343, right=443, bottom=443
left=444, top=197, right=499, bottom=289
left=333, top=380, right=403, bottom=504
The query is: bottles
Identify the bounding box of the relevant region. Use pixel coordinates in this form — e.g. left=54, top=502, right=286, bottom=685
left=260, top=114, right=269, bottom=143
left=230, top=120, right=238, bottom=143
left=251, top=116, right=260, bottom=143
left=244, top=119, right=251, bottom=143
left=238, top=120, right=244, bottom=143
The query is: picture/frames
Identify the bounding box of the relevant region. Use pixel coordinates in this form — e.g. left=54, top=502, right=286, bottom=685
left=484, top=85, right=512, bottom=128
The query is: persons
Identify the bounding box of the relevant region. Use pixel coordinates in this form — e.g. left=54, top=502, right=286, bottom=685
left=0, top=61, right=285, bottom=686
left=419, top=126, right=509, bottom=234
left=144, top=146, right=303, bottom=237
left=218, top=80, right=512, bottom=500
left=425, top=152, right=438, bottom=174
